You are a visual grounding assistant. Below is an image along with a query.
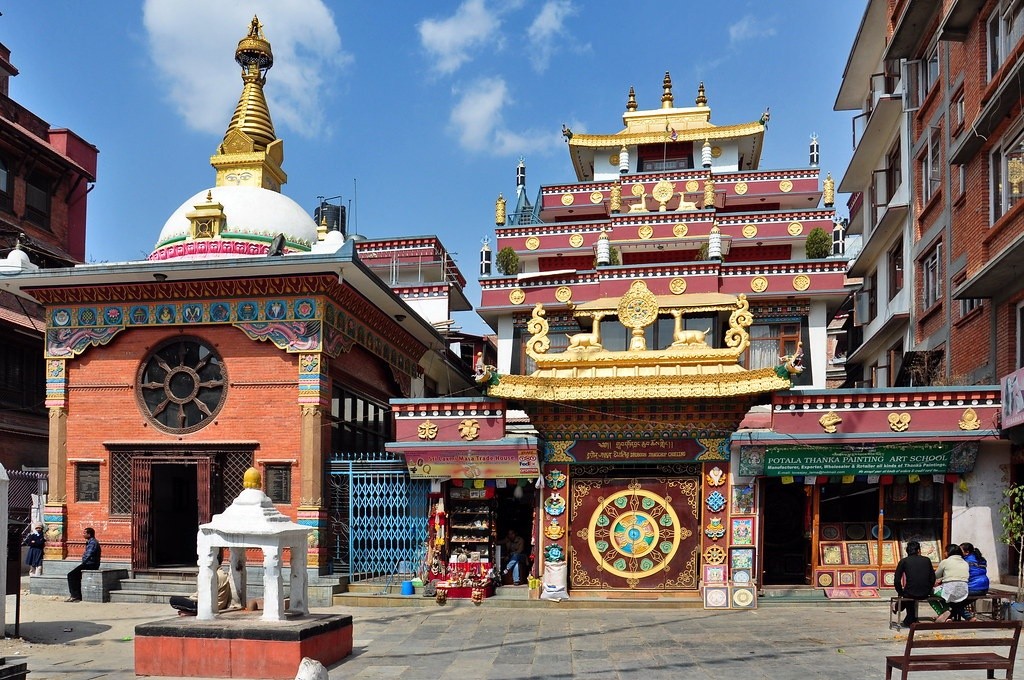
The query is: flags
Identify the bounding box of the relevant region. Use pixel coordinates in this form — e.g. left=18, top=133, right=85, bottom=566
left=665, top=120, right=679, bottom=141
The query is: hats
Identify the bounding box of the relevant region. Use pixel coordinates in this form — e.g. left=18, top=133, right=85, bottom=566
left=34, top=522, right=44, bottom=530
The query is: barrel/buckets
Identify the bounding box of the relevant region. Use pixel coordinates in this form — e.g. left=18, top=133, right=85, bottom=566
left=401, top=581, right=413, bottom=595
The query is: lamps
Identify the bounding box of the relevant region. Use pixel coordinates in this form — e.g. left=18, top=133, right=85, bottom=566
left=393, top=315, right=406, bottom=322
left=153, top=274, right=167, bottom=282
left=267, top=233, right=287, bottom=256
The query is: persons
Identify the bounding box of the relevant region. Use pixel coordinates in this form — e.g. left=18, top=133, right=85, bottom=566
left=501, top=529, right=524, bottom=586
left=927, top=540, right=989, bottom=623
left=889, top=541, right=935, bottom=627
left=64, top=528, right=101, bottom=602
left=25, top=522, right=47, bottom=576
left=1004, top=375, right=1024, bottom=418
left=170, top=547, right=230, bottom=617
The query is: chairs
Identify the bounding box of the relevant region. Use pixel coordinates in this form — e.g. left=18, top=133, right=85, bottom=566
left=504, top=561, right=524, bottom=585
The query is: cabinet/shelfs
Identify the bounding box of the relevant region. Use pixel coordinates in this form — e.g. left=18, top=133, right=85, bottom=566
left=448, top=498, right=498, bottom=571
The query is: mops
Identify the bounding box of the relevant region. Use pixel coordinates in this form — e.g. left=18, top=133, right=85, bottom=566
left=371, top=559, right=399, bottom=595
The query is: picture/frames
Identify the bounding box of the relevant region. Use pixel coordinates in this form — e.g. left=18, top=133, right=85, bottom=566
left=814, top=522, right=940, bottom=599
left=703, top=485, right=757, bottom=610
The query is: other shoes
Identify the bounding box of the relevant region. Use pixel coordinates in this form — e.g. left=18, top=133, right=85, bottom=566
left=501, top=569, right=508, bottom=575
left=892, top=598, right=896, bottom=614
left=514, top=581, right=520, bottom=585
left=64, top=596, right=79, bottom=602
left=934, top=611, right=952, bottom=623
left=968, top=617, right=977, bottom=621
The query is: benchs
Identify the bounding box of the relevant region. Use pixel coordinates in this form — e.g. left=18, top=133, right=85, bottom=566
left=886, top=620, right=1022, bottom=680
left=889, top=589, right=1016, bottom=632
